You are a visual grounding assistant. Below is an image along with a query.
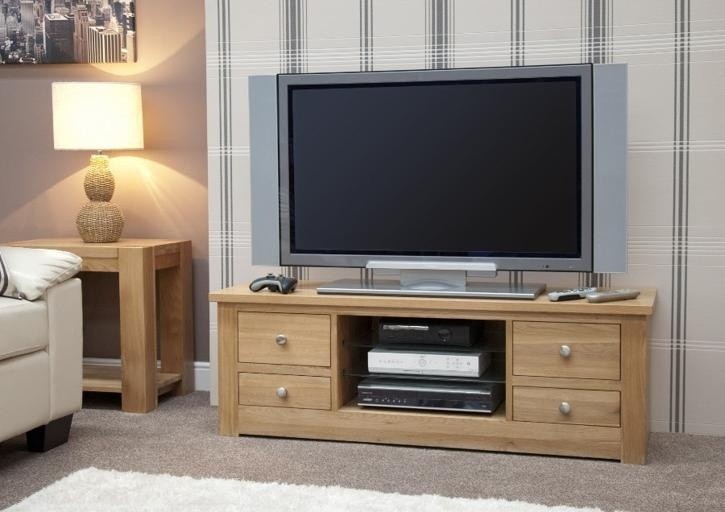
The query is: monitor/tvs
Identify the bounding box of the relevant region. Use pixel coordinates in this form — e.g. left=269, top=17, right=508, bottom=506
left=273, top=62, right=596, bottom=301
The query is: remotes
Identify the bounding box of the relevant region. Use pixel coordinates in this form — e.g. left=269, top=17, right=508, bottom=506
left=547, top=287, right=597, bottom=302
left=585, top=288, right=641, bottom=303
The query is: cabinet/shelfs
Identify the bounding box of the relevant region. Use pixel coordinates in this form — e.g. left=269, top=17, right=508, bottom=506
left=1, top=239, right=195, bottom=414
left=207, top=278, right=659, bottom=466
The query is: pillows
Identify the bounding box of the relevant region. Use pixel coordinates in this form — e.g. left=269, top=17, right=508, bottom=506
left=0, top=246, right=84, bottom=303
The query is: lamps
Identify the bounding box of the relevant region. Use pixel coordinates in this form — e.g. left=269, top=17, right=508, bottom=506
left=51, top=81, right=145, bottom=244
